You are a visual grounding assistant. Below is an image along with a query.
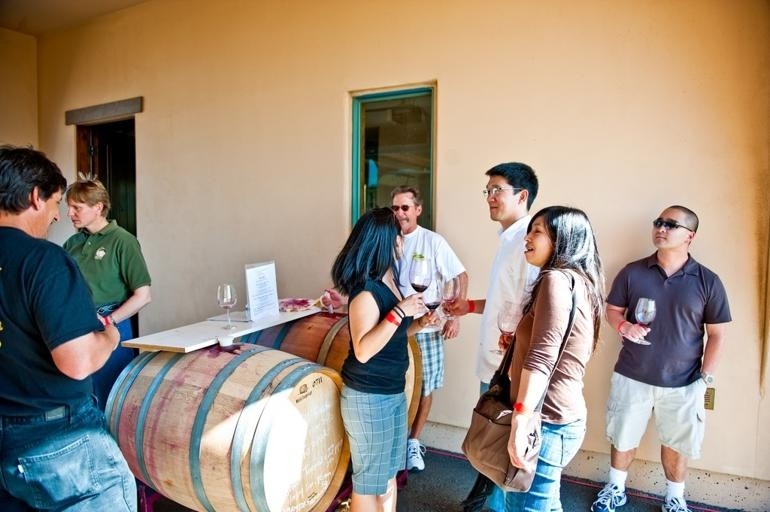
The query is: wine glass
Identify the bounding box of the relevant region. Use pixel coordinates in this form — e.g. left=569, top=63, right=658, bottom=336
left=630, top=298, right=656, bottom=346
left=441, top=275, right=461, bottom=322
left=408, top=254, right=433, bottom=312
left=216, top=283, right=238, bottom=331
left=488, top=300, right=521, bottom=356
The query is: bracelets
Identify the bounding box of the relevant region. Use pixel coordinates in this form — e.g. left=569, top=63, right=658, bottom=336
left=393, top=308, right=404, bottom=321
left=394, top=304, right=406, bottom=318
left=417, top=317, right=429, bottom=328
left=467, top=298, right=475, bottom=314
left=510, top=401, right=535, bottom=417
left=104, top=316, right=109, bottom=327
left=109, top=314, right=117, bottom=328
left=618, top=320, right=628, bottom=337
left=384, top=312, right=401, bottom=330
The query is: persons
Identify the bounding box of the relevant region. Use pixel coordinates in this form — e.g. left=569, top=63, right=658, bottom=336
left=331, top=207, right=441, bottom=512
left=0, top=141, right=140, bottom=511
left=480, top=205, right=609, bottom=512
left=318, top=184, right=469, bottom=472
left=590, top=205, right=732, bottom=512
left=443, top=161, right=547, bottom=512
left=61, top=180, right=152, bottom=413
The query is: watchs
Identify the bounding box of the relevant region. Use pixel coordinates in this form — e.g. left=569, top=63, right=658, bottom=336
left=699, top=372, right=715, bottom=386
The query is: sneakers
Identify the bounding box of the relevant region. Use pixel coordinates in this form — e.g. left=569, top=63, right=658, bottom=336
left=591, top=482, right=628, bottom=511
left=661, top=495, right=693, bottom=511
left=406, top=438, right=426, bottom=472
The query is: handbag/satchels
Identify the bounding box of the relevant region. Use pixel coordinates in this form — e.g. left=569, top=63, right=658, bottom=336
left=461, top=370, right=542, bottom=494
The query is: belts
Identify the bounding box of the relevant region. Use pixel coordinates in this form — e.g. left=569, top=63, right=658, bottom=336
left=3, top=397, right=95, bottom=425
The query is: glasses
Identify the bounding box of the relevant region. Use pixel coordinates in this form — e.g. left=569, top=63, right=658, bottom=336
left=653, top=218, right=694, bottom=233
left=483, top=187, right=523, bottom=196
left=392, top=205, right=418, bottom=211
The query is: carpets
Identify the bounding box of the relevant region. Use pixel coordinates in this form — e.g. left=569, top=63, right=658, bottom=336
left=155, top=437, right=732, bottom=512
